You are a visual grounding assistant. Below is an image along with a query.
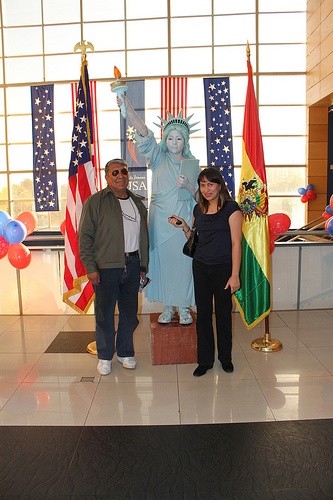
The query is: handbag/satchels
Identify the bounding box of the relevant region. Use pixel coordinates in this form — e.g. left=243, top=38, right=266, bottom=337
left=183, top=228, right=198, bottom=258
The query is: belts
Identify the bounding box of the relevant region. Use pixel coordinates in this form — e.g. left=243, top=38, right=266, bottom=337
left=123, top=250, right=138, bottom=257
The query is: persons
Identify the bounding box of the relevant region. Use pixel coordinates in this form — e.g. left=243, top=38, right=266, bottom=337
left=117, top=95, right=204, bottom=324
left=79, top=159, right=149, bottom=375
left=168, top=168, right=242, bottom=377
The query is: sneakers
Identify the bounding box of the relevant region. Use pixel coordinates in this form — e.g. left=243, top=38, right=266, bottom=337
left=97, top=359, right=112, bottom=375
left=117, top=356, right=136, bottom=369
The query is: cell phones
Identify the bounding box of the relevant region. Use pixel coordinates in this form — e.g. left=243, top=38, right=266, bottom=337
left=168, top=217, right=182, bottom=225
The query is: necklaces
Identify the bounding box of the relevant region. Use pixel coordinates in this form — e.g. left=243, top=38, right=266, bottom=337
left=167, top=152, right=181, bottom=176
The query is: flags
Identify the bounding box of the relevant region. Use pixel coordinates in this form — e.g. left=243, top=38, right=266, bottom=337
left=233, top=62, right=272, bottom=328
left=62, top=62, right=97, bottom=315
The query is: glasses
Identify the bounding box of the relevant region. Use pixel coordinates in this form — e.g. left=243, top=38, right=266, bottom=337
left=106, top=168, right=128, bottom=176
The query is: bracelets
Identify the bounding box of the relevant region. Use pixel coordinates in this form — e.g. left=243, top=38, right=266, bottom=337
left=183, top=228, right=190, bottom=233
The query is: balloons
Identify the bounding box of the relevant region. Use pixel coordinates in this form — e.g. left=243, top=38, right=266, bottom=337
left=268, top=213, right=290, bottom=254
left=0, top=210, right=36, bottom=269
left=298, top=184, right=316, bottom=203
left=322, top=194, right=333, bottom=236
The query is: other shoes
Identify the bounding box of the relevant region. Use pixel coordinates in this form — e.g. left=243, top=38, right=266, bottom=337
left=218, top=358, right=234, bottom=373
left=193, top=364, right=213, bottom=376
